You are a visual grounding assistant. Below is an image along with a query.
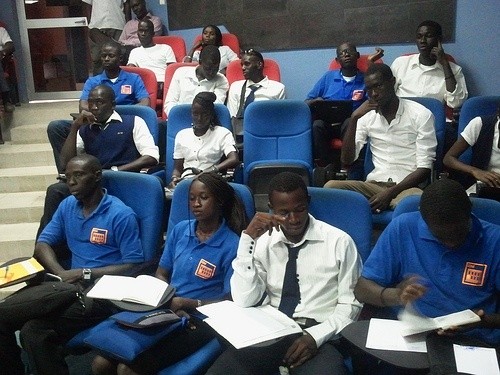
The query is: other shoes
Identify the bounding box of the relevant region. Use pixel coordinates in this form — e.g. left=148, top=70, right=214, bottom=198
left=0, top=139, right=4, bottom=144
left=4, top=102, right=15, bottom=113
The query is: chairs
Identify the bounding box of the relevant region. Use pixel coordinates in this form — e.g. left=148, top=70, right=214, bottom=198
left=69, top=33, right=500, bottom=375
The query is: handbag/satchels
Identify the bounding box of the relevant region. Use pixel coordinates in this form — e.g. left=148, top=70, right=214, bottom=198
left=82, top=309, right=189, bottom=363
left=314, top=98, right=354, bottom=123
left=0, top=281, right=87, bottom=328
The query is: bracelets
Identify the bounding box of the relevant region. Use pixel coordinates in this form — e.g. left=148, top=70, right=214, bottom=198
left=197, top=300, right=201, bottom=306
left=380, top=287, right=389, bottom=307
left=171, top=175, right=176, bottom=179
left=446, top=75, right=454, bottom=79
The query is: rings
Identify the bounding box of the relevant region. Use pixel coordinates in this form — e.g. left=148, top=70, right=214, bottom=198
left=376, top=209, right=380, bottom=212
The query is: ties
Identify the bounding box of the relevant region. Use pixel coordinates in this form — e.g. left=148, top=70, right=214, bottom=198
left=98, top=122, right=109, bottom=132
left=237, top=85, right=262, bottom=119
left=278, top=240, right=308, bottom=318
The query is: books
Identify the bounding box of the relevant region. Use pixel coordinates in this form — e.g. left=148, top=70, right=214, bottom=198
left=0, top=257, right=45, bottom=287
left=399, top=303, right=481, bottom=337
left=84, top=274, right=173, bottom=308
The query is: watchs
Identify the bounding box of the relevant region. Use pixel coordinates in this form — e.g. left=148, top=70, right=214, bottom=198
left=111, top=166, right=118, bottom=171
left=82, top=268, right=91, bottom=279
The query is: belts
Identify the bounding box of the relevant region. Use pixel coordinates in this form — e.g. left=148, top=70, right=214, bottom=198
left=293, top=318, right=319, bottom=327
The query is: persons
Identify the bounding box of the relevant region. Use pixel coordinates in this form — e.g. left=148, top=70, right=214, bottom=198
left=81, top=0, right=163, bottom=76
left=163, top=92, right=239, bottom=240
left=304, top=41, right=384, bottom=173
left=444, top=115, right=500, bottom=201
left=324, top=64, right=437, bottom=213
left=35, top=84, right=160, bottom=248
left=389, top=20, right=468, bottom=157
left=0, top=26, right=15, bottom=113
left=47, top=19, right=286, bottom=178
left=0, top=154, right=364, bottom=375
left=354, top=179, right=500, bottom=346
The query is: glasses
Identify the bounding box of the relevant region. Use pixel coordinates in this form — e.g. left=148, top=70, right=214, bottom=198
left=240, top=48, right=262, bottom=62
